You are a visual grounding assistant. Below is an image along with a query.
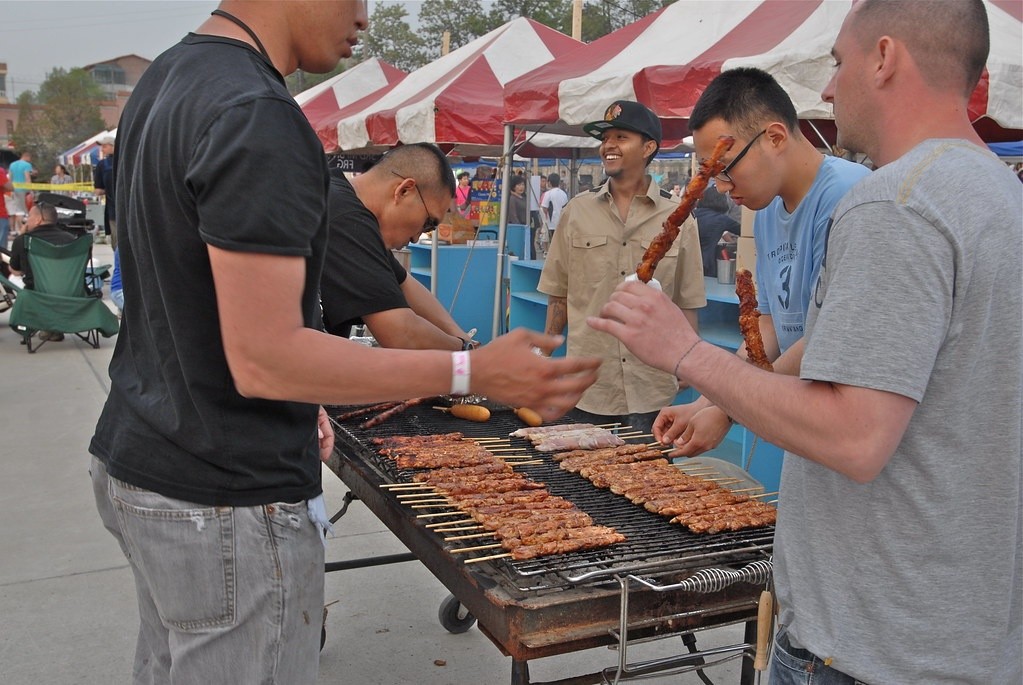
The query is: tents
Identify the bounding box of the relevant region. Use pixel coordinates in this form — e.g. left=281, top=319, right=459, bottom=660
left=293, top=0, right=1023, bottom=338
left=60, top=127, right=118, bottom=183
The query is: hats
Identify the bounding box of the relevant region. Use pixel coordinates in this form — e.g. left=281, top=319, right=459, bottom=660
left=583, top=99, right=662, bottom=155
left=96, top=137, right=114, bottom=146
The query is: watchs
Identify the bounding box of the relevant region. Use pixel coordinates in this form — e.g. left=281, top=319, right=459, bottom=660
left=458, top=337, right=474, bottom=351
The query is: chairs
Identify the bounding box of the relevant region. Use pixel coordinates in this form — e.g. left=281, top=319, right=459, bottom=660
left=12, top=235, right=105, bottom=351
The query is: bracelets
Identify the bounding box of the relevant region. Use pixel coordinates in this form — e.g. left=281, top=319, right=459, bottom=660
left=674, top=340, right=703, bottom=376
left=452, top=350, right=471, bottom=396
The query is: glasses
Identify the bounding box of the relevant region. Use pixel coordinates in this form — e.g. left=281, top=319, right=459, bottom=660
left=33, top=199, right=45, bottom=221
left=391, top=170, right=439, bottom=234
left=713, top=129, right=772, bottom=182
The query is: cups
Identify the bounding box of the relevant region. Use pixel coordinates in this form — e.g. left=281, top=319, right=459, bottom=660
left=716, top=258, right=735, bottom=284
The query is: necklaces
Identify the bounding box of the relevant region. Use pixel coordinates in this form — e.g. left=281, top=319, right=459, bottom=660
left=211, top=10, right=270, bottom=61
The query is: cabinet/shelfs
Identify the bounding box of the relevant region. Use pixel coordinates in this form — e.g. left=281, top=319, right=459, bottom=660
left=507, top=259, right=567, bottom=360
left=675, top=275, right=785, bottom=481
left=407, top=242, right=507, bottom=344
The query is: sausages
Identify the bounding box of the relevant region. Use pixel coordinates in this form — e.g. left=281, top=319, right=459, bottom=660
left=450, top=404, right=490, bottom=421
left=517, top=407, right=542, bottom=427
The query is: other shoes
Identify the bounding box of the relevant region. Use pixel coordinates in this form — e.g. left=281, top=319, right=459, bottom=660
left=38, top=331, right=64, bottom=342
left=543, top=252, right=547, bottom=258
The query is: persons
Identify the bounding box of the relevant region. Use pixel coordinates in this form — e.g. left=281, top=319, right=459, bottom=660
left=538, top=101, right=706, bottom=466
left=0, top=167, right=13, bottom=248
left=88, top=0, right=604, bottom=685
left=694, top=186, right=741, bottom=278
left=541, top=173, right=568, bottom=233
left=319, top=143, right=481, bottom=351
left=94, top=136, right=117, bottom=252
left=50, top=165, right=73, bottom=197
left=8, top=151, right=33, bottom=235
left=651, top=66, right=873, bottom=464
left=586, top=0, right=1023, bottom=685
left=455, top=172, right=471, bottom=219
left=541, top=176, right=548, bottom=195
left=8, top=202, right=76, bottom=342
left=668, top=183, right=680, bottom=196
left=720, top=191, right=742, bottom=243
left=508, top=176, right=526, bottom=224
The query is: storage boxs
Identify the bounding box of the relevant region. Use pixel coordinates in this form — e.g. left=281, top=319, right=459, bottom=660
left=736, top=204, right=756, bottom=294
